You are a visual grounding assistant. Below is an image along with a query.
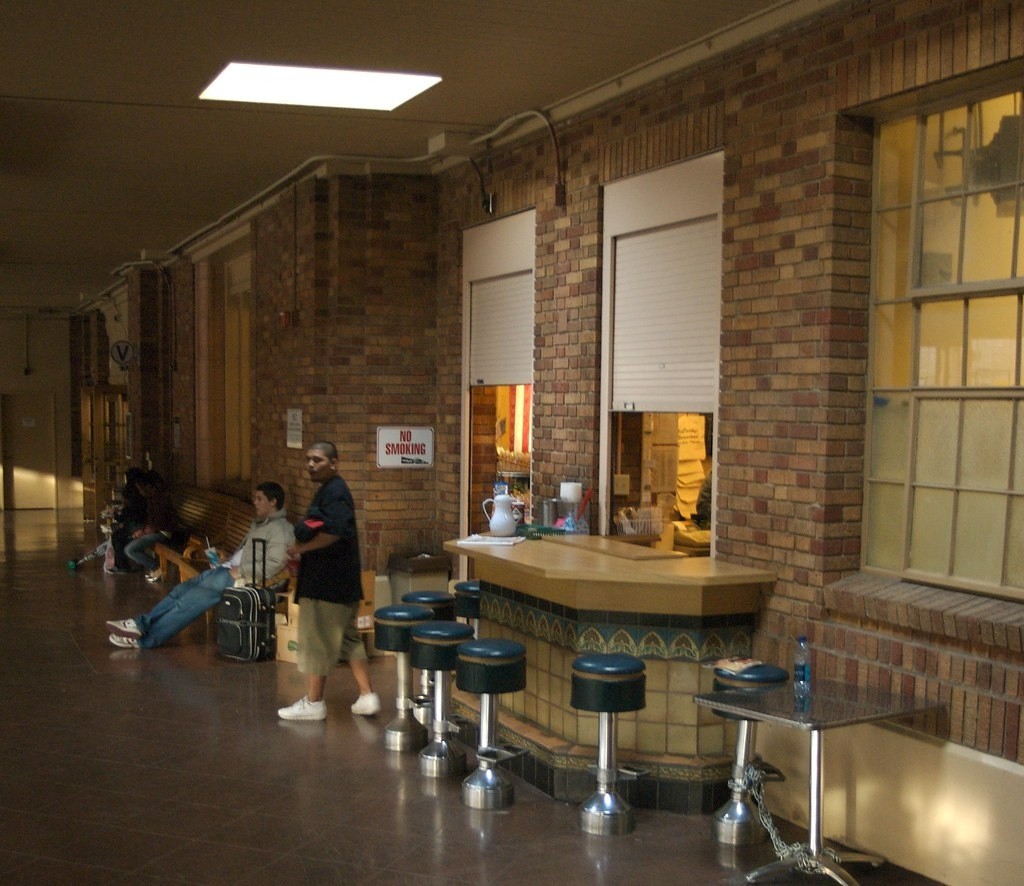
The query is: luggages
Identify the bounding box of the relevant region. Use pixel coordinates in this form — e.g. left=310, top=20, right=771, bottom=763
left=216, top=538, right=277, bottom=662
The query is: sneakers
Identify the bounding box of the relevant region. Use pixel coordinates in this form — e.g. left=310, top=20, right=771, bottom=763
left=106, top=618, right=142, bottom=639
left=351, top=692, right=382, bottom=715
left=278, top=694, right=327, bottom=720
left=109, top=633, right=141, bottom=648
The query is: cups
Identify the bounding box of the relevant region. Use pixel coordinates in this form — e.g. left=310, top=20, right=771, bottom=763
left=559, top=481, right=582, bottom=502
left=537, top=499, right=557, bottom=526
left=512, top=502, right=525, bottom=526
left=204, top=547, right=221, bottom=569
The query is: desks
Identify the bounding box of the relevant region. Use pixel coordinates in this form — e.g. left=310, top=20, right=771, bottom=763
left=693, top=678, right=948, bottom=886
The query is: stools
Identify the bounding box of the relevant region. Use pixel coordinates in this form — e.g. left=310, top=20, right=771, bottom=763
left=409, top=620, right=477, bottom=778
left=710, top=662, right=790, bottom=847
left=456, top=638, right=526, bottom=810
left=401, top=591, right=455, bottom=727
left=373, top=606, right=435, bottom=755
left=452, top=580, right=479, bottom=621
left=569, top=652, right=652, bottom=836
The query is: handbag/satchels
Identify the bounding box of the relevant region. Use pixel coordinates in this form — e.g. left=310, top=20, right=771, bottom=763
left=294, top=505, right=357, bottom=555
left=103, top=535, right=118, bottom=574
left=165, top=514, right=189, bottom=555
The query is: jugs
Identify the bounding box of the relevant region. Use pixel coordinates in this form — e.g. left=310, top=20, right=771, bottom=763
left=482, top=495, right=520, bottom=537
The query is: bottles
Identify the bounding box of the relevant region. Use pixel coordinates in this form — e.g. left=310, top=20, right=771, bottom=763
left=793, top=636, right=810, bottom=692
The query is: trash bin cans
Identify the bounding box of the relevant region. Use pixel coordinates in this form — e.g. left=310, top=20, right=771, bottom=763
left=386, top=548, right=453, bottom=606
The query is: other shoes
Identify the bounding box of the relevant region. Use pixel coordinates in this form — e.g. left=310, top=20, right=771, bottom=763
left=107, top=566, right=140, bottom=576
left=145, top=566, right=161, bottom=582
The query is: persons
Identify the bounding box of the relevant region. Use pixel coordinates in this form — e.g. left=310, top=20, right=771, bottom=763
left=696, top=470, right=712, bottom=521
left=104, top=467, right=180, bottom=583
left=105, top=482, right=296, bottom=650
left=278, top=440, right=381, bottom=720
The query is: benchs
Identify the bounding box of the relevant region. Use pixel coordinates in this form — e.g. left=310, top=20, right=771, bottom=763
left=106, top=481, right=292, bottom=624
